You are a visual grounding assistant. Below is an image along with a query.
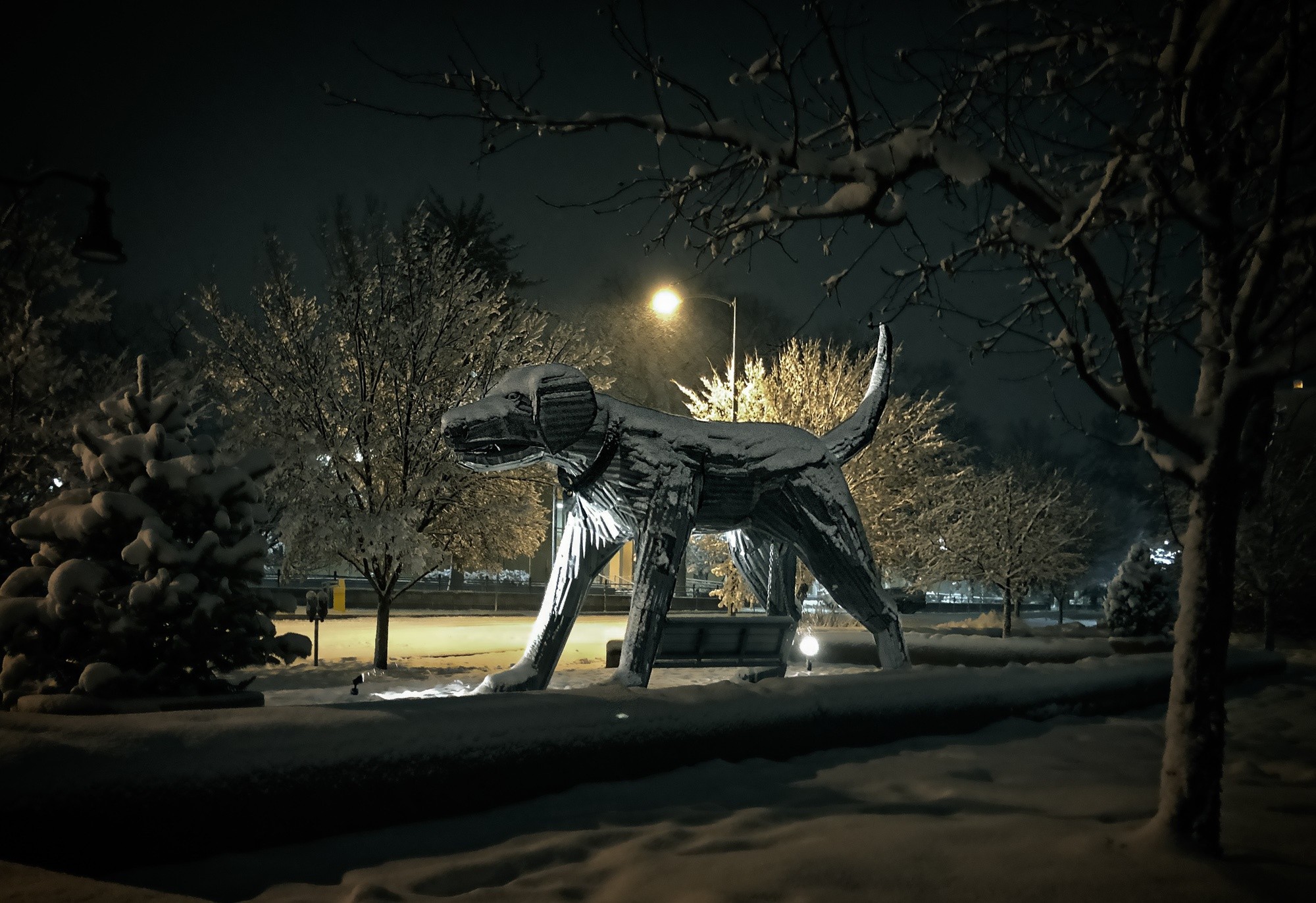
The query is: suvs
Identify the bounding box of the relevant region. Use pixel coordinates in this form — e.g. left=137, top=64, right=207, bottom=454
left=884, top=585, right=926, bottom=614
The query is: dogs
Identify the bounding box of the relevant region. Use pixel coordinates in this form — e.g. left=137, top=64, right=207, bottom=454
left=440, top=323, right=909, bottom=692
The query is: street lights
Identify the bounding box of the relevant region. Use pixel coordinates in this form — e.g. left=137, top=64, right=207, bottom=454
left=649, top=286, right=739, bottom=423
